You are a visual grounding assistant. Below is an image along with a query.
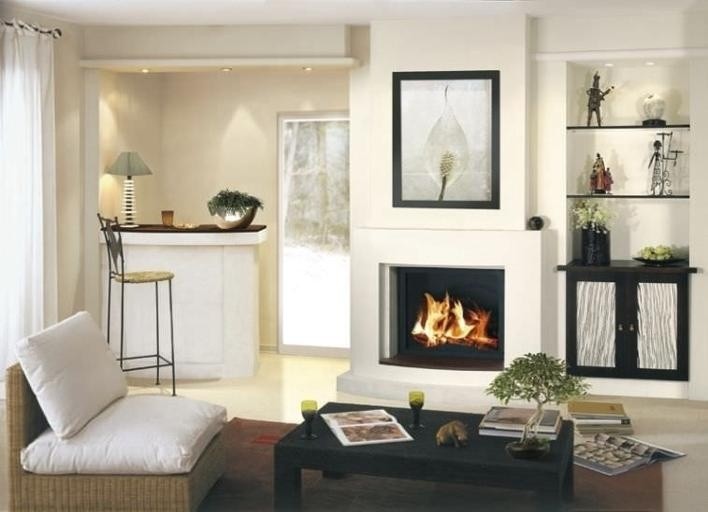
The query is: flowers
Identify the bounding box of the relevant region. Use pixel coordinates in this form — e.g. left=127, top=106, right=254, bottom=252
left=571, top=200, right=615, bottom=235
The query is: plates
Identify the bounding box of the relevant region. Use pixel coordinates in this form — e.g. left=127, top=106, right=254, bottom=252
left=631, top=255, right=684, bottom=268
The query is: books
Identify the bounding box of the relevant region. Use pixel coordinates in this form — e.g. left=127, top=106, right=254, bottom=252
left=319, top=408, right=414, bottom=447
left=482, top=407, right=560, bottom=433
left=572, top=434, right=687, bottom=476
left=568, top=401, right=635, bottom=438
left=478, top=416, right=563, bottom=441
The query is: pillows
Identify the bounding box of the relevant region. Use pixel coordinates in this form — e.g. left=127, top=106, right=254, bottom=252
left=12, top=308, right=126, bottom=439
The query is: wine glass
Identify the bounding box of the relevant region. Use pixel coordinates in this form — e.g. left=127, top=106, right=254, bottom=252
left=409, top=391, right=424, bottom=430
left=300, top=400, right=318, bottom=440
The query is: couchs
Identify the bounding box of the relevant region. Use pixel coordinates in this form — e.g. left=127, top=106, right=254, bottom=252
left=6, top=365, right=230, bottom=511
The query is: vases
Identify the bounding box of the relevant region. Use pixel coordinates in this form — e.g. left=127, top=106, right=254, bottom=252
left=581, top=228, right=612, bottom=265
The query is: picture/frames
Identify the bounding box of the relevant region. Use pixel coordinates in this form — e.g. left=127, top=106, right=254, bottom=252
left=391, top=70, right=501, bottom=210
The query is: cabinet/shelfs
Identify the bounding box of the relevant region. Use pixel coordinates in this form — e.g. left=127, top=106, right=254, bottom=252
left=567, top=124, right=691, bottom=266
left=557, top=266, right=694, bottom=381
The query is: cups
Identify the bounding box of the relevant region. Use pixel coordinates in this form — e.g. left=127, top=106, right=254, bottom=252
left=161, top=211, right=174, bottom=226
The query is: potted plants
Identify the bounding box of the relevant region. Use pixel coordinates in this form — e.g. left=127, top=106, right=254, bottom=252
left=483, top=353, right=592, bottom=459
left=207, top=188, right=263, bottom=230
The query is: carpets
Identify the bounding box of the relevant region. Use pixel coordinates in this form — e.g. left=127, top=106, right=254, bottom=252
left=194, top=418, right=663, bottom=511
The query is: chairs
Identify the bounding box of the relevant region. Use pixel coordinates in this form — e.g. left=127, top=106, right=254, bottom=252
left=97, top=213, right=176, bottom=396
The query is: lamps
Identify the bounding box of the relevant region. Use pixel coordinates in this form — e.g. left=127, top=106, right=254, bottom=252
left=105, top=151, right=153, bottom=229
